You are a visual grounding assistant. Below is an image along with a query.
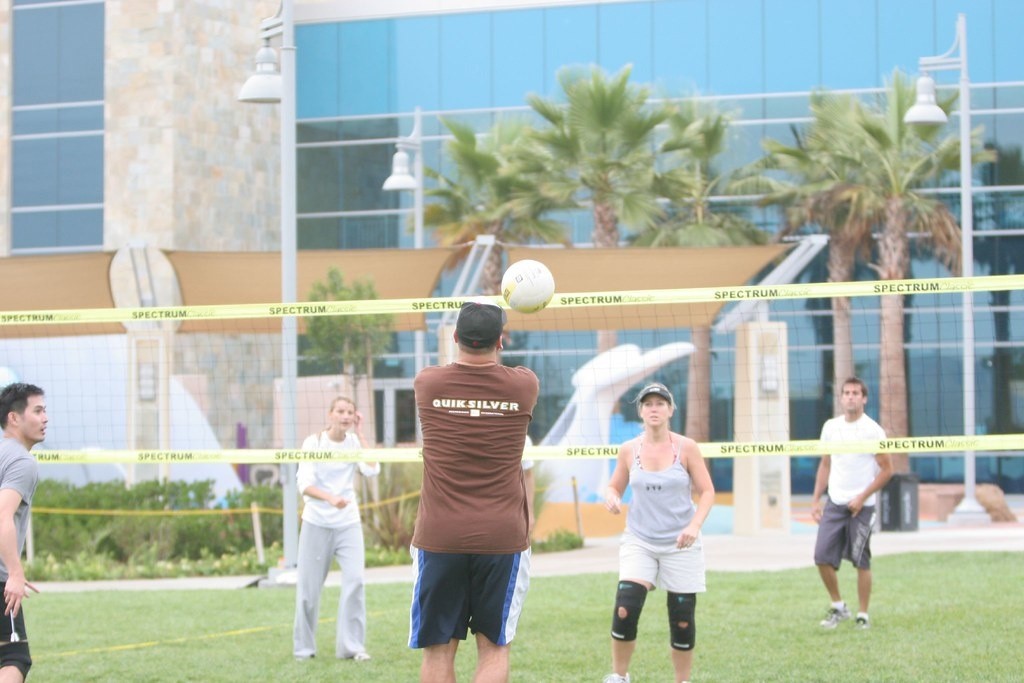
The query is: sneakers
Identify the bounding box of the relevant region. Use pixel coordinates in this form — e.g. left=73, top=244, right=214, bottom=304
left=602, top=673, right=630, bottom=683
left=854, top=618, right=869, bottom=630
left=820, top=603, right=850, bottom=629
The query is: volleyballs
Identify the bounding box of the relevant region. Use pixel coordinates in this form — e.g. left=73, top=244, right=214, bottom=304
left=501, top=260, right=556, bottom=316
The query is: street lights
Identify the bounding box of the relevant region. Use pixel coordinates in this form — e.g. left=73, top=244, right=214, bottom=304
left=235, top=0, right=303, bottom=589
left=901, top=13, right=995, bottom=527
left=379, top=108, right=429, bottom=448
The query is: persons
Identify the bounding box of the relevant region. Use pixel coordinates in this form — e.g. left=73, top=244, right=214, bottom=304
left=406, top=302, right=540, bottom=683
left=0, top=381, right=48, bottom=683
left=604, top=382, right=715, bottom=683
left=810, top=378, right=894, bottom=631
left=292, top=396, right=381, bottom=663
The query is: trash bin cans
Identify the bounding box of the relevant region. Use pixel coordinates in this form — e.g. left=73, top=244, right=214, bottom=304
left=879, top=473, right=920, bottom=532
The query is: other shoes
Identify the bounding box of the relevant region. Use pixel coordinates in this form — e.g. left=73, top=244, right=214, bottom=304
left=353, top=653, right=370, bottom=661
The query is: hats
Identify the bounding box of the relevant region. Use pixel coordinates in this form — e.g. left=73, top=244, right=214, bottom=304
left=639, top=386, right=671, bottom=404
left=456, top=299, right=503, bottom=348
